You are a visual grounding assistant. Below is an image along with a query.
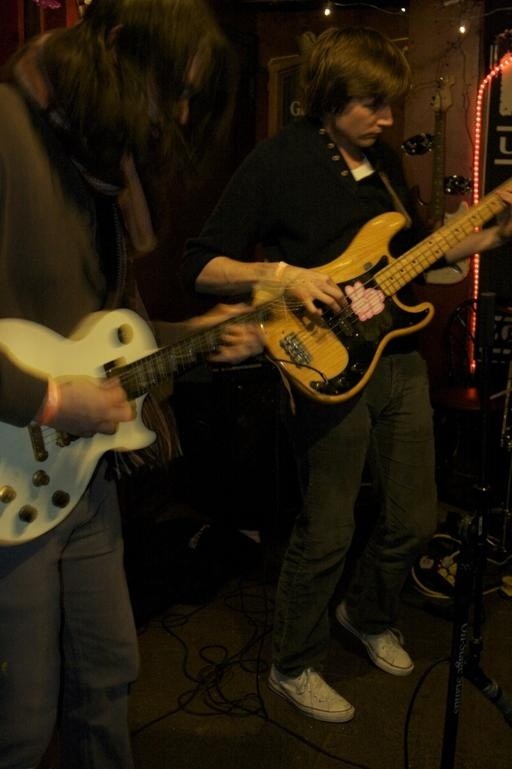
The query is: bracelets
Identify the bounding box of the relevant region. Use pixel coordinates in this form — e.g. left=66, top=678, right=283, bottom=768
left=35, top=375, right=63, bottom=426
left=272, top=259, right=289, bottom=281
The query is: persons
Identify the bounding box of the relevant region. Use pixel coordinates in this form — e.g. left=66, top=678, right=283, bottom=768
left=184, top=22, right=512, bottom=724
left=0, top=0, right=266, bottom=767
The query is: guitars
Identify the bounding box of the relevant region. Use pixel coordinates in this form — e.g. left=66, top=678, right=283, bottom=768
left=252, top=180, right=511, bottom=402
left=402, top=64, right=471, bottom=285
left=2, top=308, right=272, bottom=545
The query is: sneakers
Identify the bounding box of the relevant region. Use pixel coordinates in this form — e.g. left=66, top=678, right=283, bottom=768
left=260, top=655, right=356, bottom=725
left=333, top=592, right=418, bottom=680
